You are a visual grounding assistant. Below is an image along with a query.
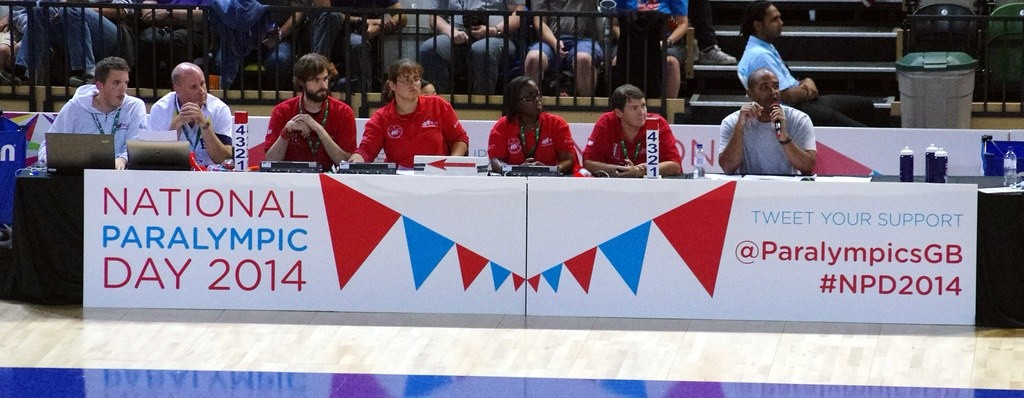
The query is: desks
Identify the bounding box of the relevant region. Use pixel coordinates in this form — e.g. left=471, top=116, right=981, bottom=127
left=12, top=162, right=1023, bottom=329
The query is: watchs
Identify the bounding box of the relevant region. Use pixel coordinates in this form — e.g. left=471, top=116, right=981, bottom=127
left=494, top=24, right=502, bottom=37
left=200, top=117, right=212, bottom=130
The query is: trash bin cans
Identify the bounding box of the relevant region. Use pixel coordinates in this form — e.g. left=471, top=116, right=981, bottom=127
left=895, top=52, right=978, bottom=129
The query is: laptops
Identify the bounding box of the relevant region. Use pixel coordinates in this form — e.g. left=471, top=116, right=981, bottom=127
left=127, top=139, right=192, bottom=171
left=45, top=133, right=115, bottom=174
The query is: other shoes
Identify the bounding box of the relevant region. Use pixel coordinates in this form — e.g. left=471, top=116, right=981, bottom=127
left=0, top=69, right=24, bottom=87
left=70, top=76, right=86, bottom=87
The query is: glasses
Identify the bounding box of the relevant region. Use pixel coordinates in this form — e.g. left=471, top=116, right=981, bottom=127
left=396, top=78, right=424, bottom=85
left=519, top=91, right=544, bottom=103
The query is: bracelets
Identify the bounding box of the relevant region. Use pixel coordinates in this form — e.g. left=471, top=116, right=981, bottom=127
left=669, top=36, right=673, bottom=47
left=278, top=28, right=282, bottom=41
left=780, top=134, right=793, bottom=146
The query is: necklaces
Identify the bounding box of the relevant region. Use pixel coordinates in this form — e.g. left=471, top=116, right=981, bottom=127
left=520, top=118, right=539, bottom=161
left=621, top=140, right=641, bottom=163
left=172, top=93, right=202, bottom=155
left=91, top=109, right=121, bottom=136
left=299, top=95, right=329, bottom=155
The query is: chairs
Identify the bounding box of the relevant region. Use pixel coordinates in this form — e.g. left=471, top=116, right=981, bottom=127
left=905, top=4, right=1023, bottom=91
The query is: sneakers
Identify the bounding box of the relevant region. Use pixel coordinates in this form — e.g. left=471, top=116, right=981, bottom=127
left=699, top=45, right=736, bottom=64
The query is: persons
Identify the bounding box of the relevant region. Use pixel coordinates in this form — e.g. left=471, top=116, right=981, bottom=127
left=346, top=59, right=469, bottom=162
left=582, top=83, right=683, bottom=178
left=265, top=53, right=356, bottom=169
left=737, top=0, right=875, bottom=127
left=0, top=0, right=737, bottom=89
left=489, top=75, right=581, bottom=177
left=150, top=62, right=234, bottom=165
left=39, top=56, right=149, bottom=172
left=719, top=68, right=818, bottom=177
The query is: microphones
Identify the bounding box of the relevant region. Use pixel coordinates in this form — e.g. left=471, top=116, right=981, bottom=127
left=770, top=102, right=782, bottom=140
left=594, top=170, right=610, bottom=178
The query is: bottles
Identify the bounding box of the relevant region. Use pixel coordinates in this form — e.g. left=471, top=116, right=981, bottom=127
left=693, top=144, right=707, bottom=178
left=1004, top=146, right=1018, bottom=187
left=925, top=144, right=948, bottom=183
left=900, top=146, right=914, bottom=182
